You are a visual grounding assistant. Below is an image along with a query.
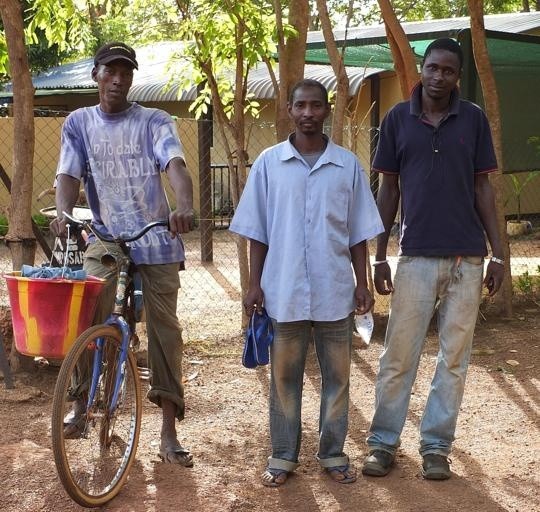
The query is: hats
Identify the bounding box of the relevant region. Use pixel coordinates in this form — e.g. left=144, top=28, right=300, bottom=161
left=94, top=41, right=139, bottom=71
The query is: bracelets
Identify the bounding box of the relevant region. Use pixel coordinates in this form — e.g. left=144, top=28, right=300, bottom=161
left=372, top=260, right=388, bottom=266
left=491, top=256, right=505, bottom=266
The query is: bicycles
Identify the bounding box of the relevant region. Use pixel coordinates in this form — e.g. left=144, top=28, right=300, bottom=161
left=50, top=208, right=199, bottom=508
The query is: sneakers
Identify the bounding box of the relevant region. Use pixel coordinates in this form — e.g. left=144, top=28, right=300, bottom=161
left=361, top=447, right=396, bottom=477
left=421, top=452, right=452, bottom=480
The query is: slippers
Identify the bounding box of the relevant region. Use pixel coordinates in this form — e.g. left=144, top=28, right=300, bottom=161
left=325, top=463, right=357, bottom=484
left=260, top=467, right=292, bottom=486
left=241, top=307, right=274, bottom=368
left=157, top=439, right=193, bottom=466
left=62, top=409, right=90, bottom=435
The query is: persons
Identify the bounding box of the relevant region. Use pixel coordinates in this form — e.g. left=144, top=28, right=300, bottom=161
left=229, top=79, right=386, bottom=487
left=362, top=37, right=506, bottom=480
left=49, top=41, right=197, bottom=467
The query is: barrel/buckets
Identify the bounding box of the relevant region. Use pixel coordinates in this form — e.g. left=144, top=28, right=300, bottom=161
left=211, top=182, right=223, bottom=214
left=2, top=270, right=106, bottom=360
